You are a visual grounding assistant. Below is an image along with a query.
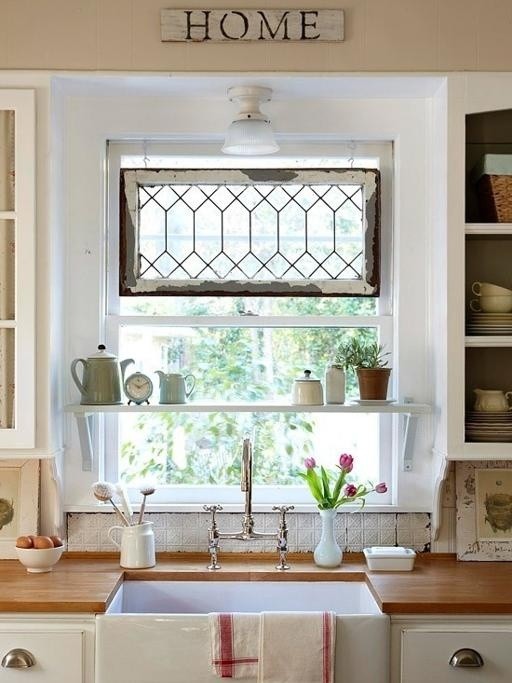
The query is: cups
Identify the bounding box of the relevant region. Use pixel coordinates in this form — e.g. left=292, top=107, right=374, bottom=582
left=469, top=280, right=512, bottom=313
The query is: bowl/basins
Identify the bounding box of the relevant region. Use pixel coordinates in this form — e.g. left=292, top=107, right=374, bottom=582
left=13, top=542, right=65, bottom=572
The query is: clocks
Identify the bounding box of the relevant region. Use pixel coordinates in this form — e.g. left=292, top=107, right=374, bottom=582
left=123, top=372, right=152, bottom=406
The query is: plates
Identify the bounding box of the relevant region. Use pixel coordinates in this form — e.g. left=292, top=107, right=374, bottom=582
left=466, top=311, right=512, bottom=335
left=465, top=409, right=512, bottom=442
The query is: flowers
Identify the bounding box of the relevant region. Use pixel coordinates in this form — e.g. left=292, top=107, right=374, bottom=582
left=295, top=451, right=390, bottom=515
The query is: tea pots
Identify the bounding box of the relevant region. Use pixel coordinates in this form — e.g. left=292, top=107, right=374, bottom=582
left=70, top=342, right=136, bottom=407
left=154, top=369, right=197, bottom=405
left=107, top=520, right=157, bottom=569
left=474, top=387, right=512, bottom=411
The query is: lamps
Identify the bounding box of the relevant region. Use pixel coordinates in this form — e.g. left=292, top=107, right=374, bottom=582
left=220, top=84, right=282, bottom=157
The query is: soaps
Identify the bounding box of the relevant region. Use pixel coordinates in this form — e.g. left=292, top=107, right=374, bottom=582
left=371, top=546, right=407, bottom=555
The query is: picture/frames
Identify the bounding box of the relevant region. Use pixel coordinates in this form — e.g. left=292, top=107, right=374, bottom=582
left=451, top=460, right=512, bottom=560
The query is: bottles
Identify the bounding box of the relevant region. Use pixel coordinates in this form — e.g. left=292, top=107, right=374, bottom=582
left=289, top=368, right=324, bottom=406
left=323, top=362, right=348, bottom=404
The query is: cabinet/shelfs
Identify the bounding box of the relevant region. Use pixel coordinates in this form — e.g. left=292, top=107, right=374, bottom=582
left=0, top=85, right=38, bottom=451
left=396, top=614, right=510, bottom=683
left=434, top=67, right=512, bottom=460
left=1, top=615, right=93, bottom=683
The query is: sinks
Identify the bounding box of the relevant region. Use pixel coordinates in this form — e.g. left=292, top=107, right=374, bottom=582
left=94, top=579, right=391, bottom=683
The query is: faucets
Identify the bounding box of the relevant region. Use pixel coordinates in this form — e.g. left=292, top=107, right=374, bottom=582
left=240, top=437, right=255, bottom=533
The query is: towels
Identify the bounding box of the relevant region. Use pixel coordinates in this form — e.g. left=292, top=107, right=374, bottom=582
left=209, top=608, right=261, bottom=680
left=260, top=608, right=339, bottom=683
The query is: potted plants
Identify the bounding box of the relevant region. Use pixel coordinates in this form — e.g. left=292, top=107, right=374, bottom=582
left=333, top=332, right=394, bottom=402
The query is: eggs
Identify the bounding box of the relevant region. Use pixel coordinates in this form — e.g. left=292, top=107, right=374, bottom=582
left=16, top=535, right=63, bottom=549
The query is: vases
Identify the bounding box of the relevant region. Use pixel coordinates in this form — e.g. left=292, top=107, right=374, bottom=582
left=313, top=505, right=345, bottom=569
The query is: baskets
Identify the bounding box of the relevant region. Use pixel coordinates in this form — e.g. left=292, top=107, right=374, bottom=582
left=478, top=173, right=512, bottom=223
